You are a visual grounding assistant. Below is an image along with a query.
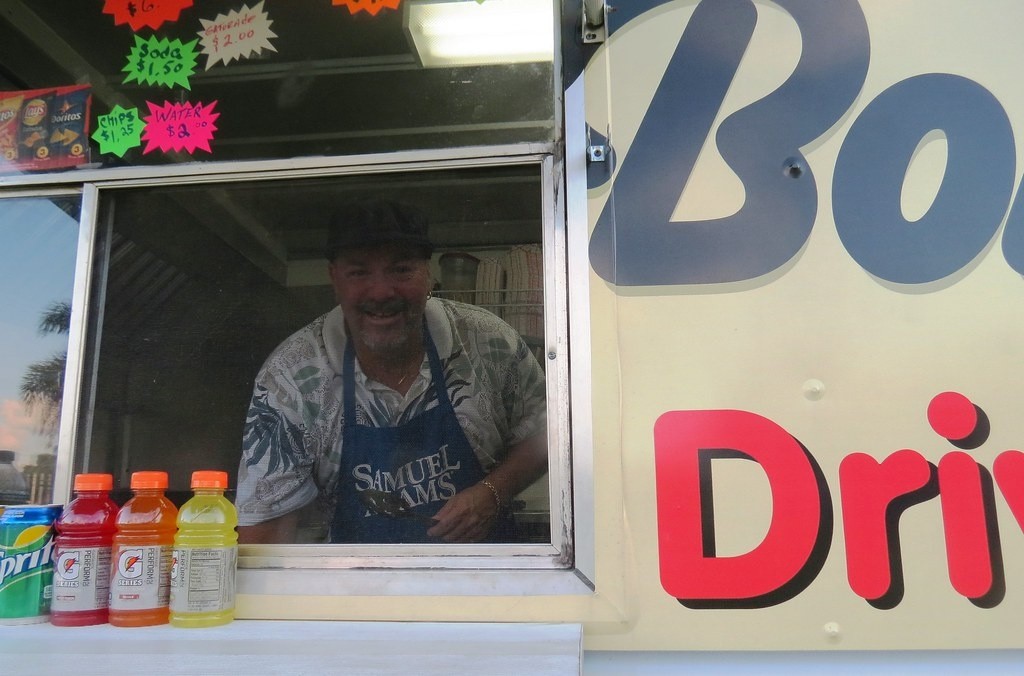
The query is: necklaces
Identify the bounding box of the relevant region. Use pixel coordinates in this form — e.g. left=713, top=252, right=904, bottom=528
left=390, top=375, right=406, bottom=391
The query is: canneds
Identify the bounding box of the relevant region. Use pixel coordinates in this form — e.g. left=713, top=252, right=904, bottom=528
left=0, top=503, right=63, bottom=626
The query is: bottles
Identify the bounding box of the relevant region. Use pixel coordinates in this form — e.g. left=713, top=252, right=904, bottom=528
left=107, top=470, right=179, bottom=628
left=49, top=472, right=119, bottom=627
left=167, top=469, right=238, bottom=629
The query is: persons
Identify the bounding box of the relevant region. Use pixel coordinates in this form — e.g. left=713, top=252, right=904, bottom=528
left=234, top=199, right=547, bottom=544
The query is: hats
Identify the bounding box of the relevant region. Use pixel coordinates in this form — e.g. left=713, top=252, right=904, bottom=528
left=323, top=188, right=433, bottom=264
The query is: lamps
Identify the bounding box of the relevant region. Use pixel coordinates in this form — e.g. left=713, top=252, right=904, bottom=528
left=402, top=0, right=554, bottom=69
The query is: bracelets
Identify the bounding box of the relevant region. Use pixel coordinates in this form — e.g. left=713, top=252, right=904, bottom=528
left=479, top=479, right=500, bottom=519
